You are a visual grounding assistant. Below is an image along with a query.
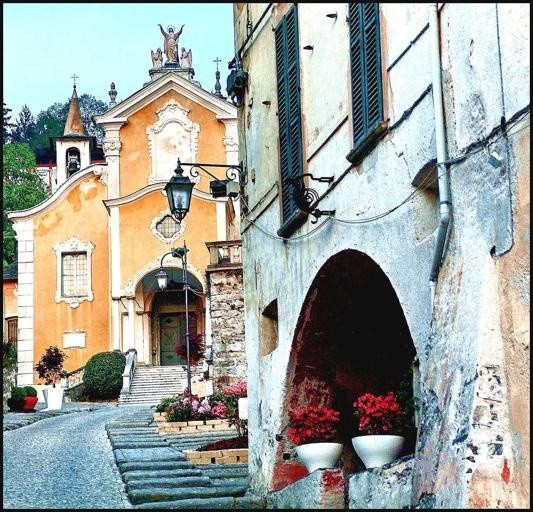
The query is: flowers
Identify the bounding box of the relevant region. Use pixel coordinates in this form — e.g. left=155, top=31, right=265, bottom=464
left=286, top=405, right=341, bottom=445
left=351, top=391, right=403, bottom=434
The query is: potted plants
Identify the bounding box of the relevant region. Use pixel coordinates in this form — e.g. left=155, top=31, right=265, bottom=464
left=7, top=386, right=25, bottom=412
left=175, top=328, right=207, bottom=366
left=35, top=346, right=69, bottom=411
left=23, top=386, right=38, bottom=412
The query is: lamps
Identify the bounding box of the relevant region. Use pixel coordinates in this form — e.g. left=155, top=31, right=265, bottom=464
left=162, top=157, right=247, bottom=224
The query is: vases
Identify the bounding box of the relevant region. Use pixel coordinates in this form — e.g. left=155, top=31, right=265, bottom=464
left=294, top=442, right=344, bottom=474
left=351, top=434, right=405, bottom=469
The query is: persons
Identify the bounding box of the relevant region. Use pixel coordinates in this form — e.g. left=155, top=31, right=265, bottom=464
left=179, top=47, right=192, bottom=66
left=156, top=23, right=186, bottom=62
left=151, top=48, right=164, bottom=67
